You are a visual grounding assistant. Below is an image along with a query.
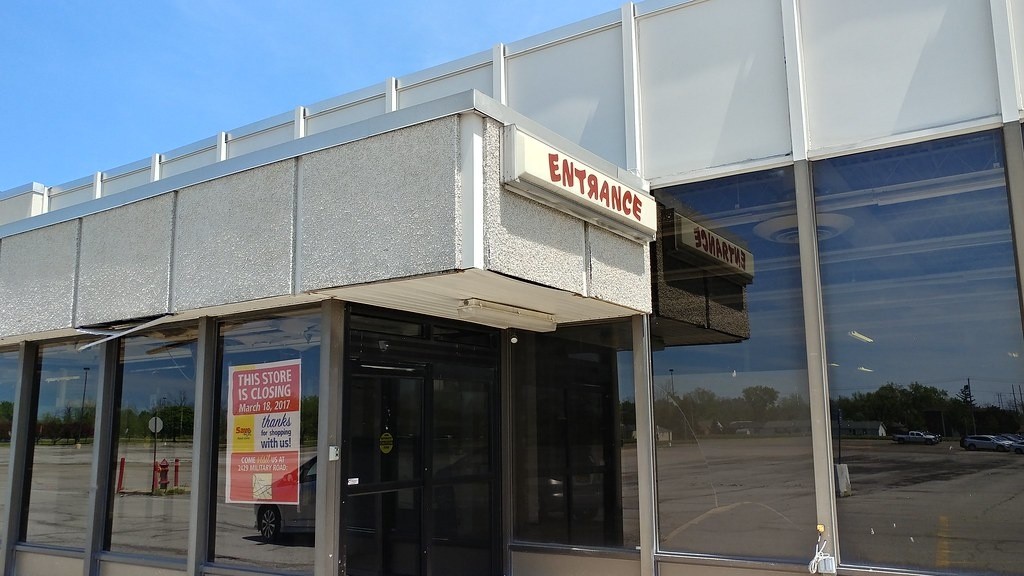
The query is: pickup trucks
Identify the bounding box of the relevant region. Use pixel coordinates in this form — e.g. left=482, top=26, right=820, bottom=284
left=893, top=431, right=936, bottom=445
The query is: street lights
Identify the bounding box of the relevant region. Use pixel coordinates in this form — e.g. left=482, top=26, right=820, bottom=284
left=669, top=369, right=675, bottom=395
left=78, top=368, right=90, bottom=442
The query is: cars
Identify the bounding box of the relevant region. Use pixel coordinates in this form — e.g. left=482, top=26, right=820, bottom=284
left=921, top=431, right=942, bottom=444
left=434, top=435, right=605, bottom=520
left=960, top=434, right=1024, bottom=452
left=1010, top=441, right=1024, bottom=454
left=255, top=433, right=522, bottom=541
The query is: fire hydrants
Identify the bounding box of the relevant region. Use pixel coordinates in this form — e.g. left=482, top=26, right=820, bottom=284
left=157, top=459, right=170, bottom=489
